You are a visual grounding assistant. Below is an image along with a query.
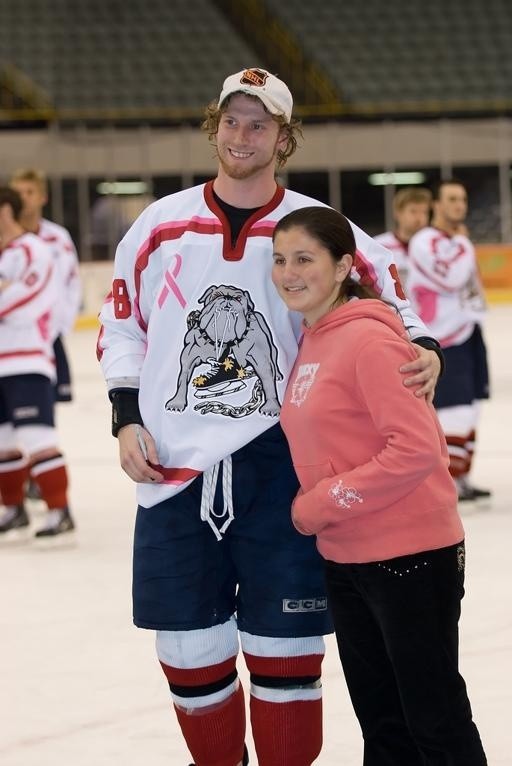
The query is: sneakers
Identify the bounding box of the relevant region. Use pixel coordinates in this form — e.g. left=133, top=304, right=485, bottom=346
left=457, top=488, right=489, bottom=502
left=36, top=507, right=75, bottom=536
left=0, top=504, right=28, bottom=531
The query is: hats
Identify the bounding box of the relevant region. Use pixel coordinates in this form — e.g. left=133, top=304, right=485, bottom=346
left=217, top=68, right=293, bottom=124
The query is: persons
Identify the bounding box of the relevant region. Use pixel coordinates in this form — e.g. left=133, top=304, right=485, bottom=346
left=6, top=169, right=82, bottom=405
left=367, top=183, right=436, bottom=311
left=96, top=64, right=446, bottom=764
left=0, top=182, right=77, bottom=545
left=268, top=205, right=493, bottom=763
left=407, top=177, right=493, bottom=507
